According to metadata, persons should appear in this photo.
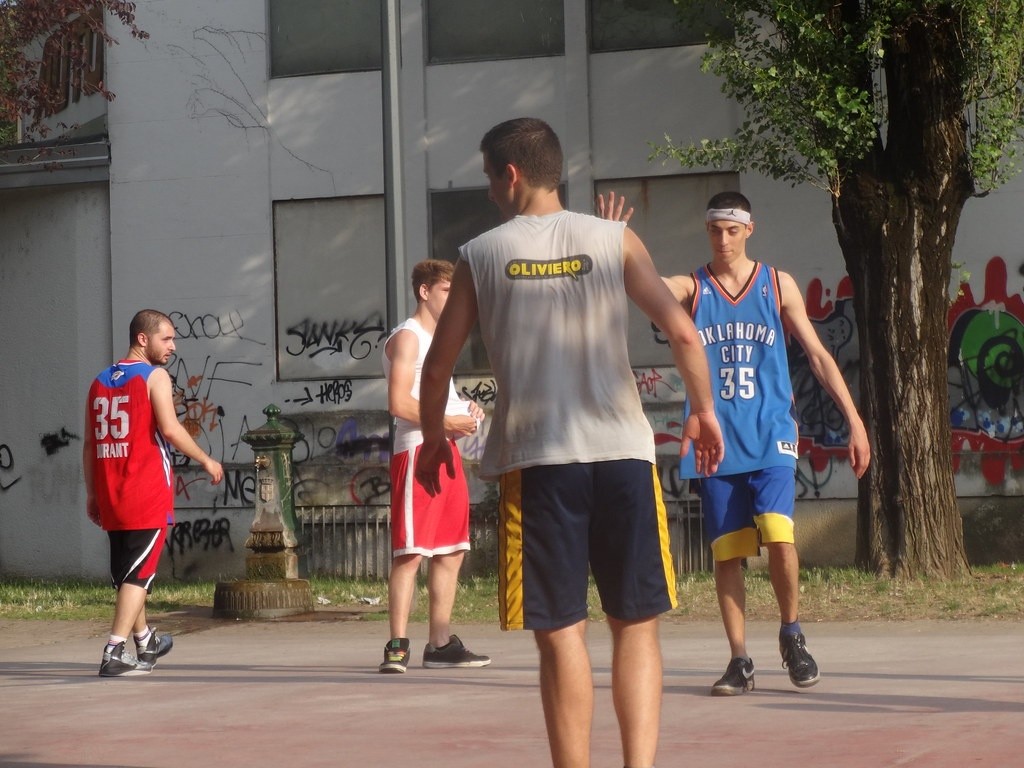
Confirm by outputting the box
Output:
[598,189,871,698]
[412,117,726,767]
[379,258,492,675]
[81,308,225,677]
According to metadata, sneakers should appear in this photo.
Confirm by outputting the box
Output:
[712,633,821,696]
[378,634,491,675]
[101,627,174,675]
